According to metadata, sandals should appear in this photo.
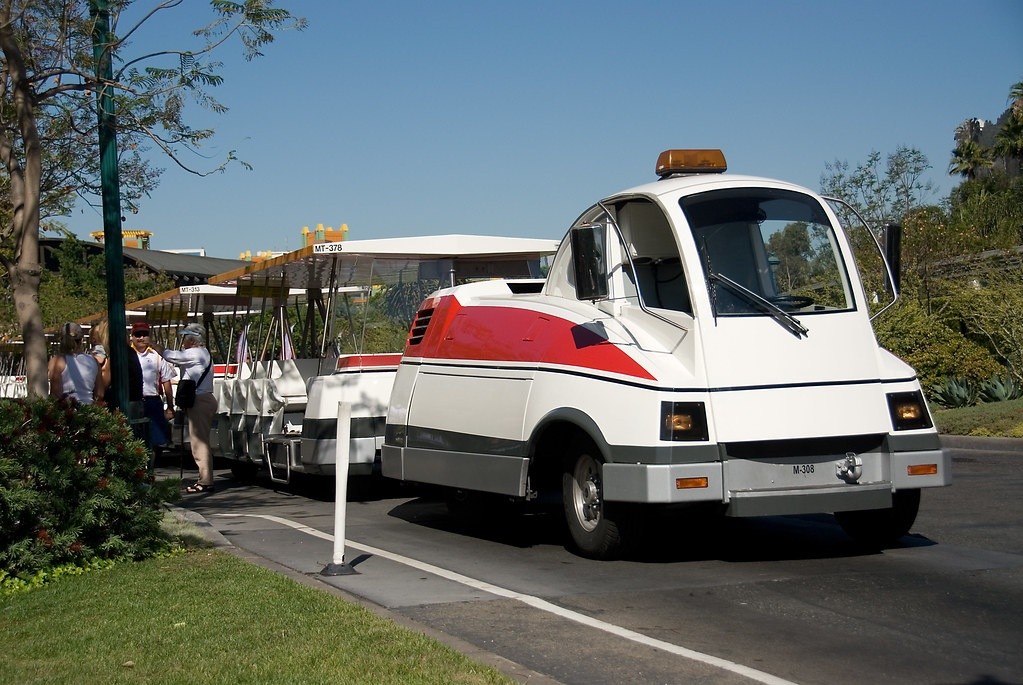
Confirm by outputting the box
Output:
[186,483,216,492]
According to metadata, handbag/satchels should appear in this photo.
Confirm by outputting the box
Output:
[175,380,196,408]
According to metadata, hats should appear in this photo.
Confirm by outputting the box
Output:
[177,322,206,337]
[63,322,83,340]
[131,322,150,337]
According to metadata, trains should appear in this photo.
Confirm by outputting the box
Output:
[0,141,954,561]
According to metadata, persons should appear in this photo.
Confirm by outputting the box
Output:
[49,321,99,408]
[132,322,177,468]
[143,322,219,493]
[88,320,143,441]
